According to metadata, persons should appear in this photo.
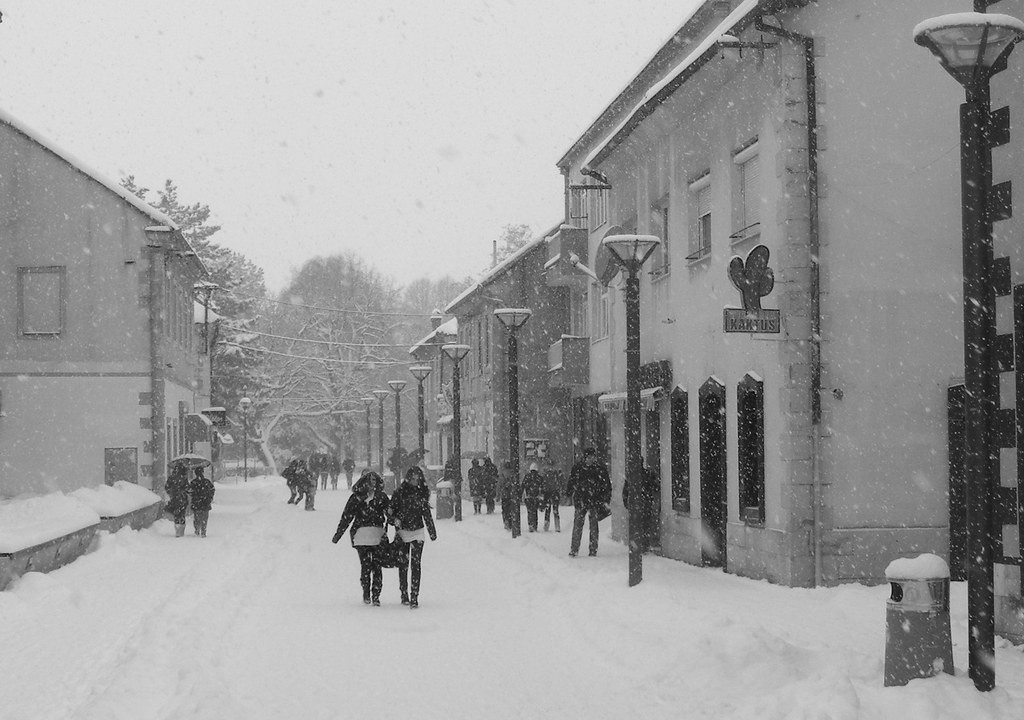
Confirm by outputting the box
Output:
[342,453,355,491]
[482,457,499,514]
[388,465,436,608]
[332,466,401,606]
[540,459,566,532]
[295,457,340,511]
[468,459,482,514]
[496,460,513,531]
[565,447,612,557]
[623,455,661,554]
[188,466,215,537]
[520,463,544,531]
[164,463,190,537]
[281,460,297,504]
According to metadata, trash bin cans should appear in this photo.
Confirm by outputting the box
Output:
[885,576,954,687]
[436,478,454,520]
[382,472,395,495]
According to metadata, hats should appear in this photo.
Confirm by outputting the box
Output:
[289,456,297,462]
[530,462,538,472]
[584,447,595,454]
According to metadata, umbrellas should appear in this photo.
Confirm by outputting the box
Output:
[166,453,211,471]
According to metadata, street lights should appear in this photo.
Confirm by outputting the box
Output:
[914,14,1024,687]
[602,235,662,585]
[373,391,389,474]
[494,309,534,539]
[442,345,470,520]
[410,367,432,474]
[388,381,407,488]
[240,397,250,482]
[360,398,374,468]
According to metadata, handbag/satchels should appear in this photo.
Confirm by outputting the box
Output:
[374,520,408,569]
[282,468,291,478]
[165,497,184,515]
[594,501,611,521]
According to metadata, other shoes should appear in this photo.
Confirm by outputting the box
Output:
[363,592,370,604]
[410,596,418,608]
[589,550,597,557]
[195,528,207,538]
[372,596,380,606]
[401,594,410,605]
[569,549,578,556]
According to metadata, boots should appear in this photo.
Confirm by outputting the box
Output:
[176,525,186,537]
